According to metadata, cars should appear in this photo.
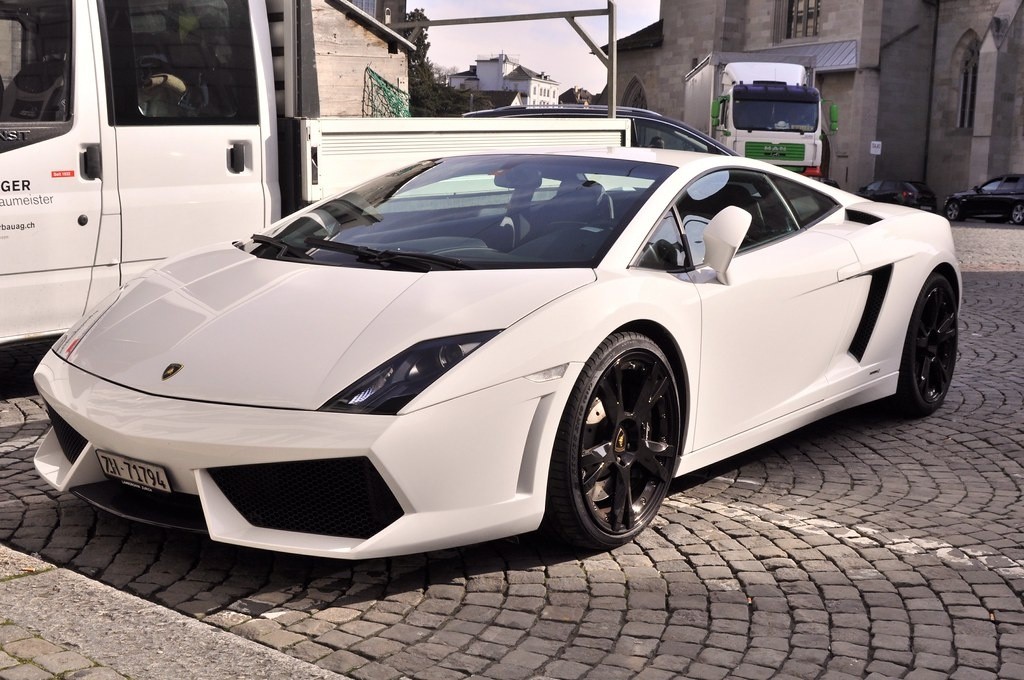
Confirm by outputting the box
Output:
[943,173,1024,224]
[853,179,938,214]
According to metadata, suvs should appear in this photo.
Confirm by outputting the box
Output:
[459,101,745,190]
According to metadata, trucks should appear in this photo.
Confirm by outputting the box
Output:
[682,51,841,190]
[0,0,633,352]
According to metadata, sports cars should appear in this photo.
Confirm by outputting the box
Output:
[31,147,963,564]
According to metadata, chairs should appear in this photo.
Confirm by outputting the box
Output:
[676,182,765,240]
[0,63,65,122]
[535,181,614,233]
